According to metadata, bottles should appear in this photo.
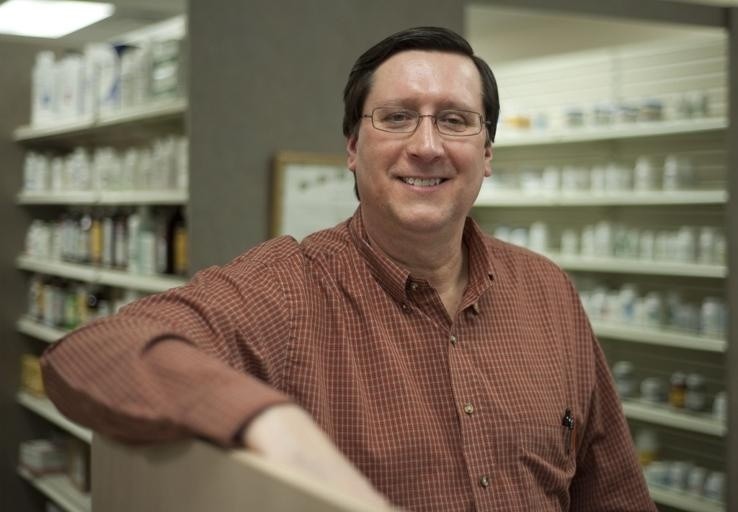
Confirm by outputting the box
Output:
[478,152,732,501]
[15,38,187,330]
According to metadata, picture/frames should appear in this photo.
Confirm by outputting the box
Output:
[269,150,358,251]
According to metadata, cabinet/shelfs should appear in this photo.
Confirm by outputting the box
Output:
[12,38,207,512]
[481,112,737,512]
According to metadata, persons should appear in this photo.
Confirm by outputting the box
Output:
[41,26,658,512]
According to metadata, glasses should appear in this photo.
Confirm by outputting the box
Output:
[359,106,492,137]
